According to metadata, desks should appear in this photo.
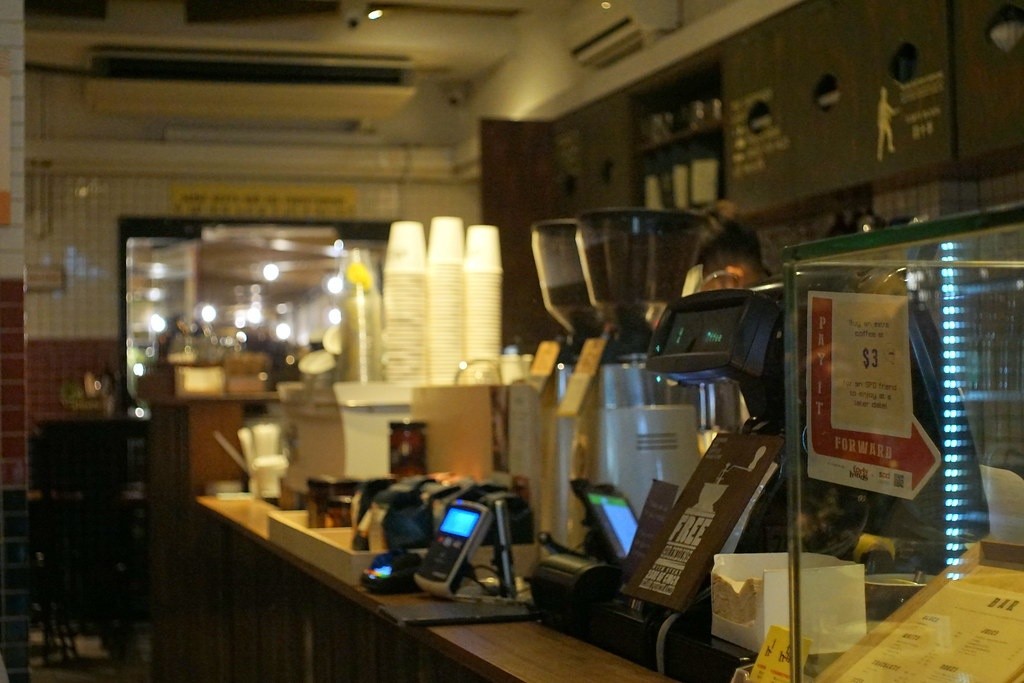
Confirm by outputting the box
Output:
[28,480,145,503]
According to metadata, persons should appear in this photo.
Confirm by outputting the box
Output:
[698,224,766,293]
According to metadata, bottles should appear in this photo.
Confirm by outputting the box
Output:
[386,422,427,478]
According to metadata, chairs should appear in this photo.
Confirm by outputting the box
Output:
[35,458,128,664]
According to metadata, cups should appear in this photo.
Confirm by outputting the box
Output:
[383,217,503,383]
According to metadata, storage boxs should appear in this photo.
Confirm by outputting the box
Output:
[782,205,1024,682]
